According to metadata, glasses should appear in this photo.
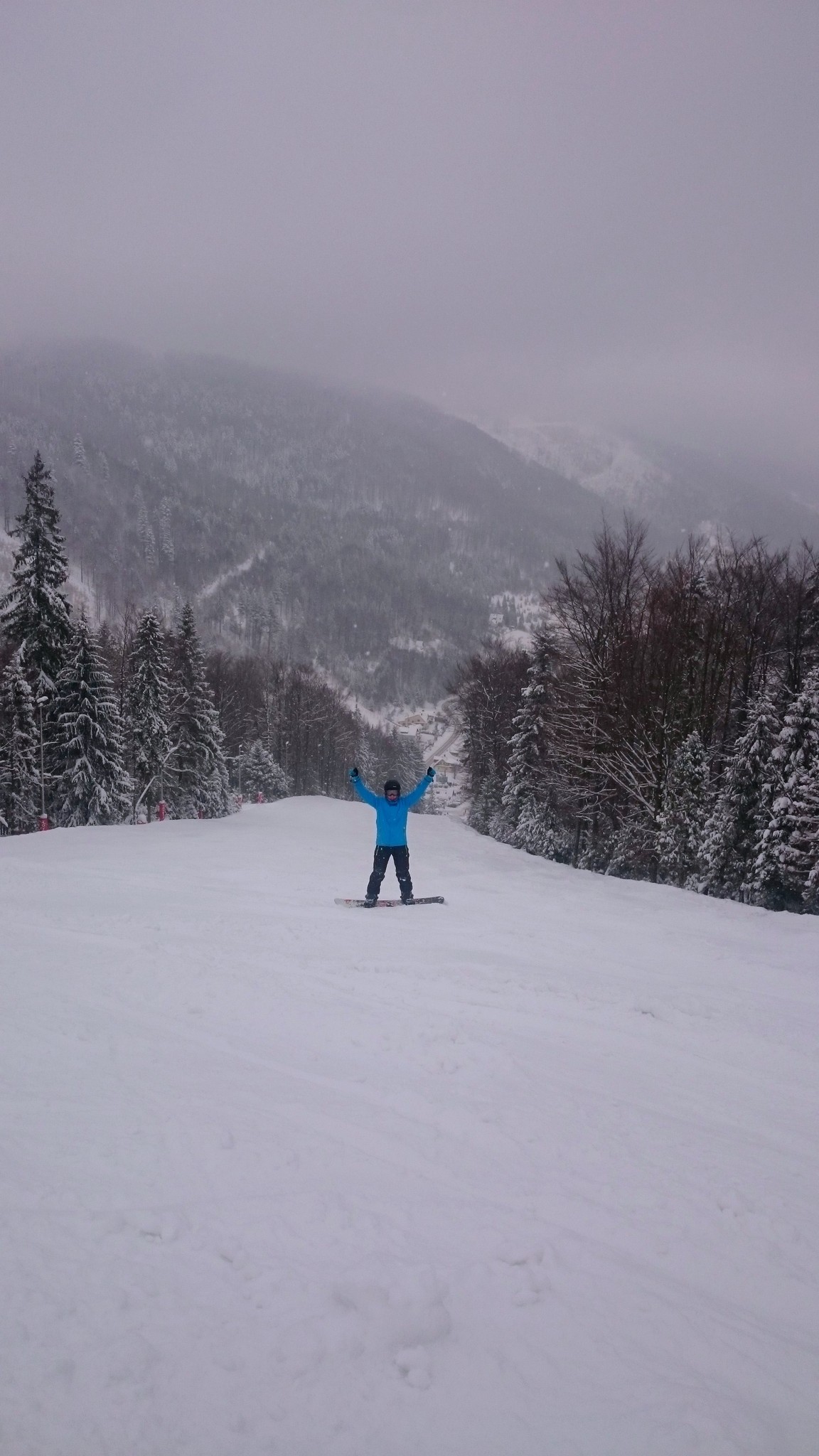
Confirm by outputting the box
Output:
[386,790,399,796]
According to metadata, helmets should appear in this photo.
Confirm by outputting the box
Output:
[384,780,400,790]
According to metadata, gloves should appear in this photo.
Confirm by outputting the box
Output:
[350,768,358,777]
[427,766,436,778]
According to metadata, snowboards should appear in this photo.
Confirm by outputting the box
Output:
[334,896,444,909]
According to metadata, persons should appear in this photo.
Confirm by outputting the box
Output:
[348,766,436,908]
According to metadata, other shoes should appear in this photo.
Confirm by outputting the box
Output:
[364,892,378,908]
[401,893,415,904]
